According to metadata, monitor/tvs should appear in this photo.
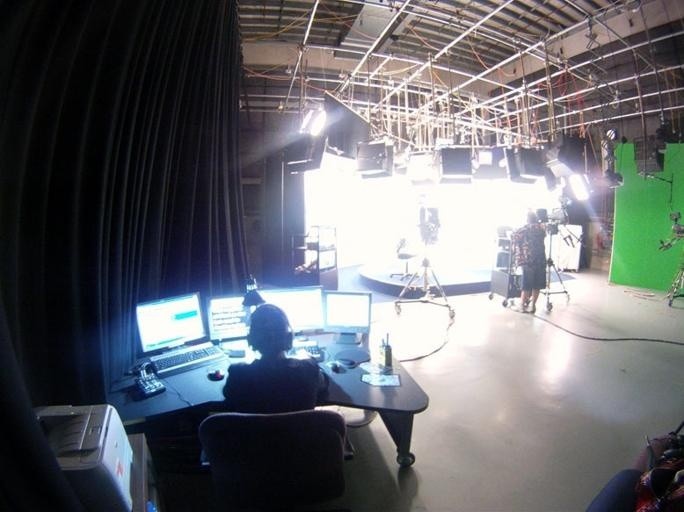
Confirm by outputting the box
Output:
[132,289,208,356]
[250,284,325,341]
[207,292,255,341]
[324,291,372,345]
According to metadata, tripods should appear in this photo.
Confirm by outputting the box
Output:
[539,231,571,312]
[392,245,457,318]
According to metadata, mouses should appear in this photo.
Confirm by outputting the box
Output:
[327,360,341,373]
[209,366,225,381]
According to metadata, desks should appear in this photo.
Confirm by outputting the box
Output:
[126,431,162,512]
[106,333,428,466]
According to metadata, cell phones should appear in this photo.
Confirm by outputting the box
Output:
[228,347,246,359]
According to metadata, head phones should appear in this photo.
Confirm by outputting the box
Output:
[245,303,293,353]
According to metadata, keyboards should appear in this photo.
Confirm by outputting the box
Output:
[148,340,224,376]
[289,343,324,363]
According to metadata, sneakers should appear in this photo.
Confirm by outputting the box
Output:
[510,305,524,312]
[524,307,534,313]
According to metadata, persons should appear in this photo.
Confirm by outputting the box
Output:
[220,303,331,413]
[509,210,549,315]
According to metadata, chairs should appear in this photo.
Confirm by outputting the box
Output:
[196,405,355,511]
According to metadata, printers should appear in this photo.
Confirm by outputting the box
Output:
[35,403,137,511]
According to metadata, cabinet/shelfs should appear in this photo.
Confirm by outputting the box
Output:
[289,224,338,292]
